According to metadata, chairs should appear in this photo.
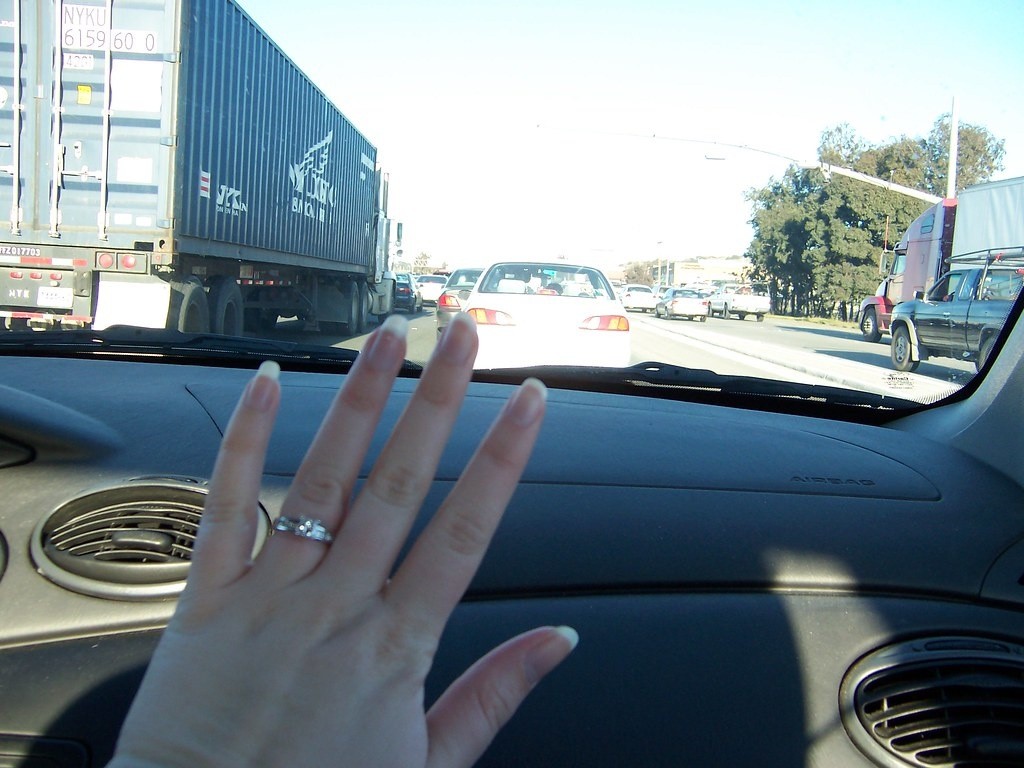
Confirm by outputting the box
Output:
[497,279,527,294]
[564,285,595,296]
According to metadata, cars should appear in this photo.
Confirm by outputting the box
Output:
[558,281,592,297]
[395,272,423,313]
[461,260,631,369]
[416,270,452,304]
[619,285,657,313]
[656,286,670,299]
[655,288,708,322]
[436,268,484,340]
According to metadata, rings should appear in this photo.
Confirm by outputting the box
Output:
[273,515,334,543]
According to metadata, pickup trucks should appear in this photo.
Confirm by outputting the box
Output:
[707,285,770,322]
[889,246,1024,373]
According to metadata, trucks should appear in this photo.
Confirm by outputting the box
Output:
[858,176,1024,343]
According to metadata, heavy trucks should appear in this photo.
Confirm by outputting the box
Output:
[0,0,402,336]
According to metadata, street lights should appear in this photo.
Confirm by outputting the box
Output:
[658,241,662,284]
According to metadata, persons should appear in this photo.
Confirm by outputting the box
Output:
[107,313,579,768]
[943,295,953,301]
[547,282,563,295]
[525,273,536,293]
